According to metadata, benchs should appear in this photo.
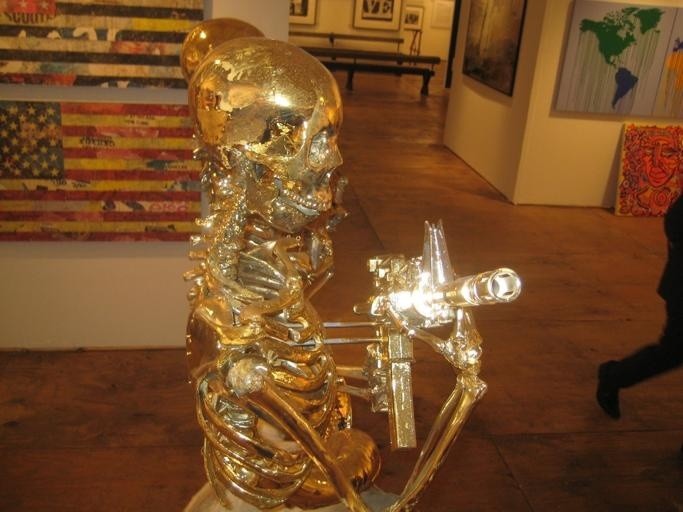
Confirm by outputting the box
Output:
[290,30,441,96]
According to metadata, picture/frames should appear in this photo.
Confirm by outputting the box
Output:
[461,0,528,97]
[289,0,318,25]
[353,0,402,31]
[404,6,424,30]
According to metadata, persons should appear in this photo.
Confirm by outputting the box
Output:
[594,192,682,417]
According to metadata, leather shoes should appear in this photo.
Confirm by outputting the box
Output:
[596,360,621,420]
